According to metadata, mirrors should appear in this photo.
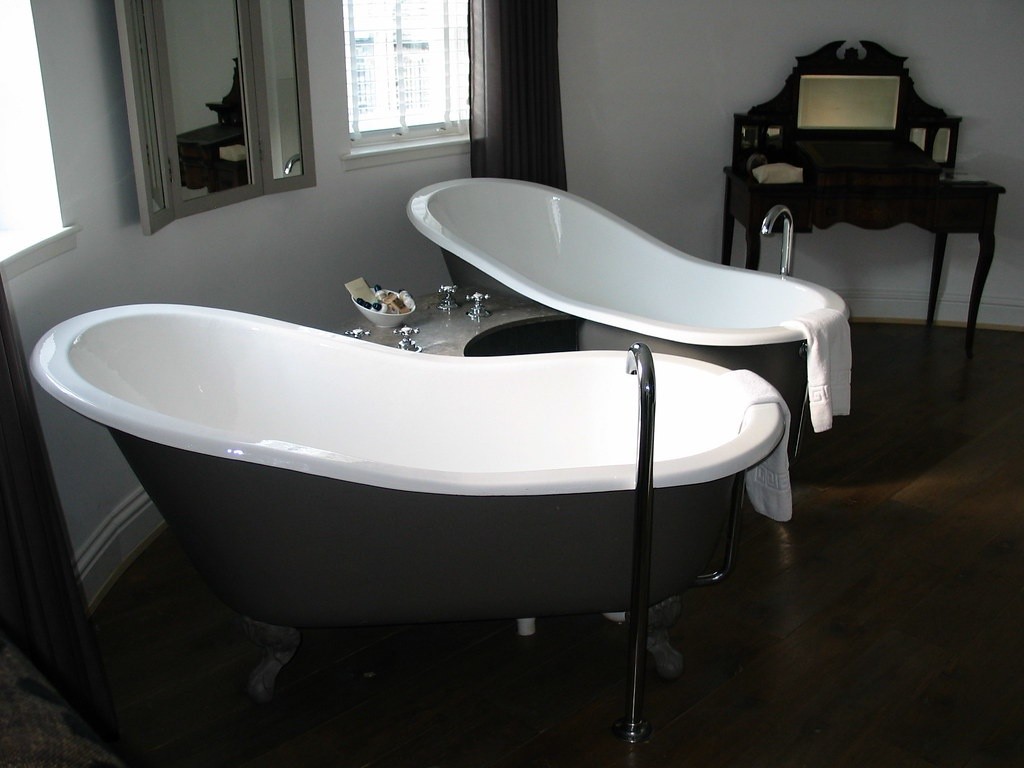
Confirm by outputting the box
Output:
[114,0,318,234]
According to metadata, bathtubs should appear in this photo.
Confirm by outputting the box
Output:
[30,305,785,707]
[406,178,852,469]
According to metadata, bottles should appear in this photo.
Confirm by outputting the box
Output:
[357,298,388,313]
[374,285,386,302]
[398,289,414,309]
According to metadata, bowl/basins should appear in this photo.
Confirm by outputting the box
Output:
[351,288,416,328]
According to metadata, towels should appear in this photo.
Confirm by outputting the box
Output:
[781,306,853,432]
[685,369,794,521]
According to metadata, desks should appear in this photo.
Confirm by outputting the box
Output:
[721,39,1007,361]
[171,54,251,196]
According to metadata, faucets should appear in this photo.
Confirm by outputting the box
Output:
[613,341,651,742]
[762,206,794,279]
